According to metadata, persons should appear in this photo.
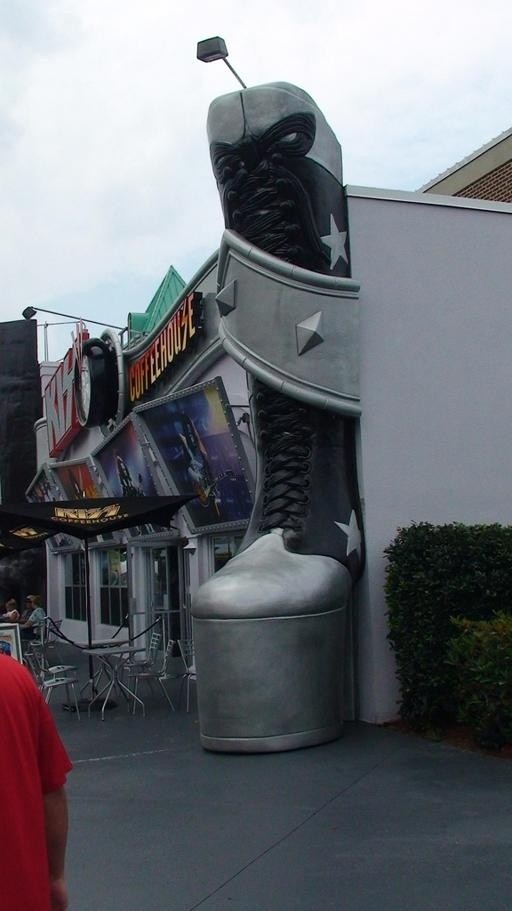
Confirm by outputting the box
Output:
[1,593,47,651]
[0,648,72,908]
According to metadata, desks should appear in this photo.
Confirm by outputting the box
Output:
[0,617,40,630]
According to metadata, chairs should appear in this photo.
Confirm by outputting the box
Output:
[24,619,197,722]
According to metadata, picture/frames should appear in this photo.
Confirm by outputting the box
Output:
[0,623,23,665]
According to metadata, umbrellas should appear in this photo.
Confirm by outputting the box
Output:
[0,488,192,718]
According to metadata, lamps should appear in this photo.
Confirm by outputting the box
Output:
[22,305,123,331]
[197,36,245,86]
